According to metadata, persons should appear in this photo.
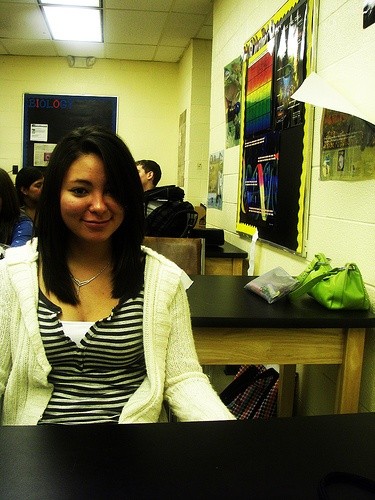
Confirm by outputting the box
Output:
[0,124,238,426]
[0,166,45,260]
[135,159,184,238]
[244,19,276,62]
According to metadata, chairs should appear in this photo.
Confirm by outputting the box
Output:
[141,237,205,275]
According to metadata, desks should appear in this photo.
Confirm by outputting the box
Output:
[197,236,248,275]
[0,413,375,500]
[186,276,375,414]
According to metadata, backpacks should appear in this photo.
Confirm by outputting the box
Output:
[142,201,199,238]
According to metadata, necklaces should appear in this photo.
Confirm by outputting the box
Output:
[68,258,111,295]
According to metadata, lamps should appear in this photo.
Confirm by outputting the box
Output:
[66,55,96,66]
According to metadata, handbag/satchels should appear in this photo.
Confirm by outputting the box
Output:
[219,363,301,420]
[288,252,371,310]
